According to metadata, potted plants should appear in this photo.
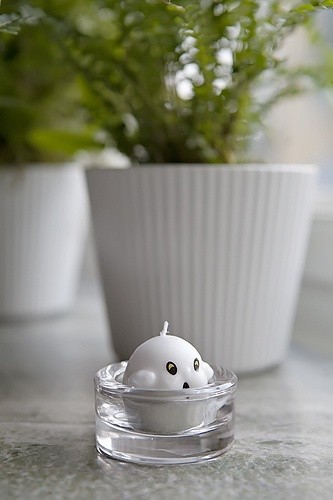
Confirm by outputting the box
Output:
[0,0,186,320]
[0,0,333,374]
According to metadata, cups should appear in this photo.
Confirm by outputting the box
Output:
[85,164,318,375]
[0,161,91,323]
